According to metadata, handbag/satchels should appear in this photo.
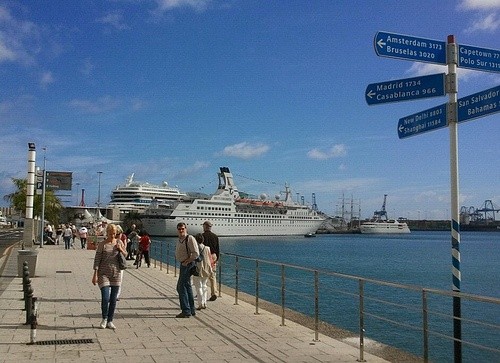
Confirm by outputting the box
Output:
[190,266,199,276]
[118,250,127,270]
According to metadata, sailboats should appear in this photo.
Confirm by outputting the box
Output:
[315,193,365,234]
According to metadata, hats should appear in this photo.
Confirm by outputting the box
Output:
[202,221,212,226]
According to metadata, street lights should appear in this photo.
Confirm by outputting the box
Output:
[96,171,103,207]
[40,146,47,249]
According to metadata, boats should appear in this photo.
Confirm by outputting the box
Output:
[251,198,264,206]
[264,200,276,207]
[359,219,411,234]
[234,199,251,205]
[304,232,316,237]
[276,201,285,208]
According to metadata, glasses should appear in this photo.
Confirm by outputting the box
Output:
[178,228,185,230]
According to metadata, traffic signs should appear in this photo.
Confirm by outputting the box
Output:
[397,101,449,140]
[365,71,447,106]
[373,30,448,66]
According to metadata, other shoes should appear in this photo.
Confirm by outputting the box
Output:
[176,313,189,317]
[107,322,116,329]
[116,298,119,301]
[197,305,206,310]
[100,319,107,328]
[210,296,216,301]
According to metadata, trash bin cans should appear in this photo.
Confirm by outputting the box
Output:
[16,250,39,278]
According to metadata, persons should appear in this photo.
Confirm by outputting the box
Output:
[175,223,199,318]
[121,224,150,269]
[99,222,107,227]
[45,222,77,250]
[92,224,127,329]
[109,225,123,300]
[203,221,220,301]
[194,234,214,310]
[79,224,88,248]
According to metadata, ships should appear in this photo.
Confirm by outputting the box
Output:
[106,173,186,214]
[126,167,326,236]
